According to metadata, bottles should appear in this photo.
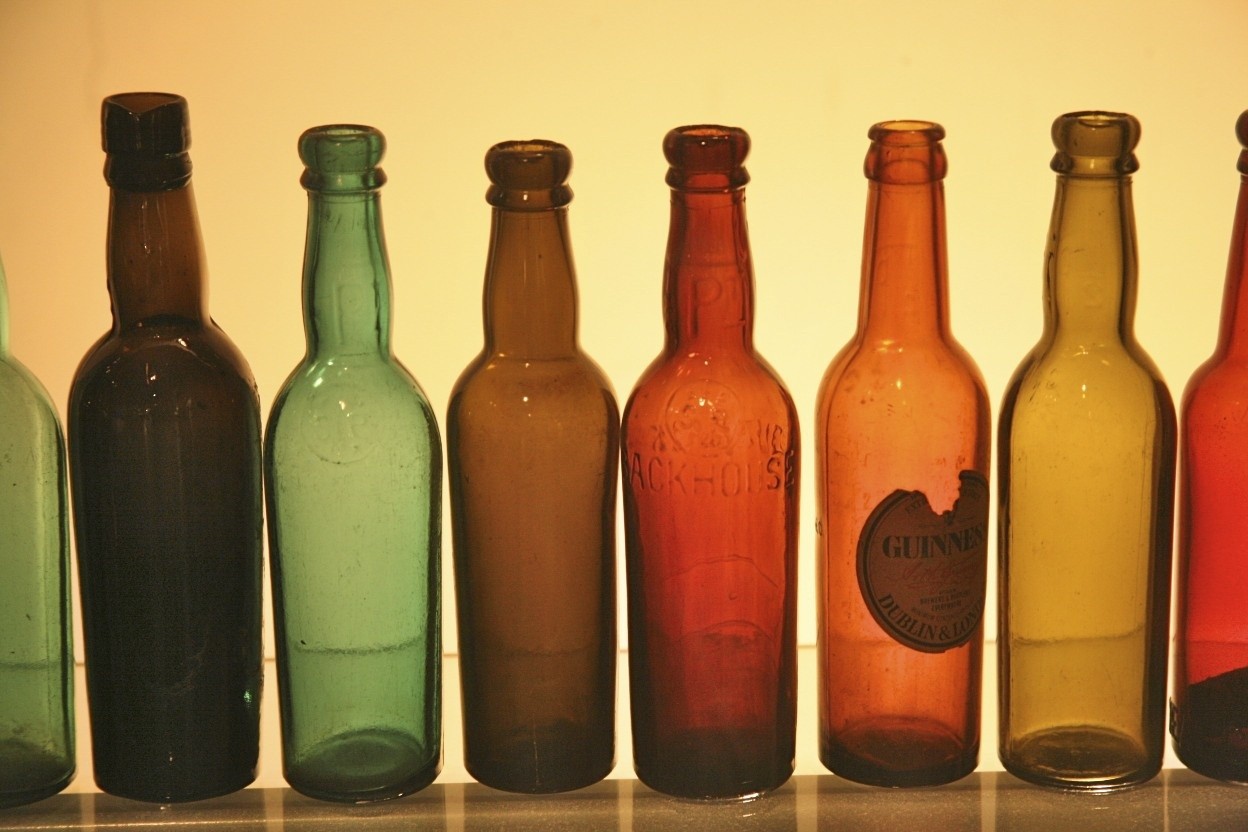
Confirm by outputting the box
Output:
[1169,109,1247,790]
[812,117,991,792]
[0,256,74,809]
[446,139,622,794]
[66,95,264,806]
[622,123,803,803]
[996,109,1178,798]
[264,122,444,809]
